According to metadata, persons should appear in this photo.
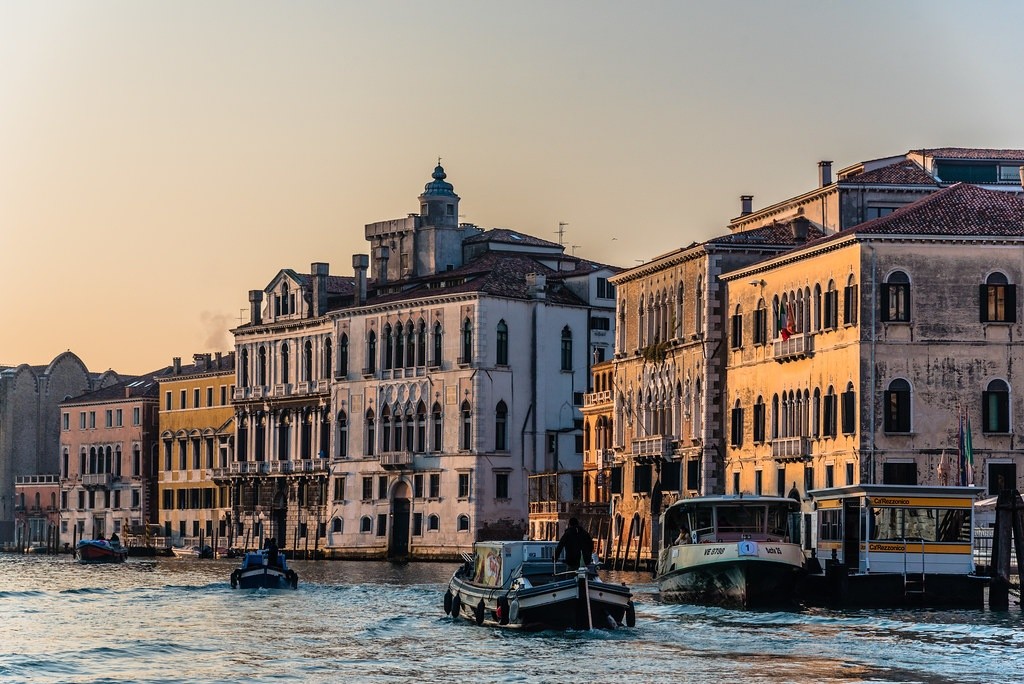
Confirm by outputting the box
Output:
[95,532,105,540]
[108,532,119,541]
[673,525,692,546]
[261,538,279,565]
[552,517,594,580]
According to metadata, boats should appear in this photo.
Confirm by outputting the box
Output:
[231,548,298,590]
[77,540,128,564]
[24,544,47,555]
[443,540,635,636]
[649,492,803,613]
[171,546,237,560]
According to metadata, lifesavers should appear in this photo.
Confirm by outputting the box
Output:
[496,595,509,625]
[230,569,241,587]
[286,569,298,589]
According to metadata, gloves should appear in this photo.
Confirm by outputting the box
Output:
[551,556,558,563]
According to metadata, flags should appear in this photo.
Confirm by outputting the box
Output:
[780,303,796,342]
[958,410,975,486]
